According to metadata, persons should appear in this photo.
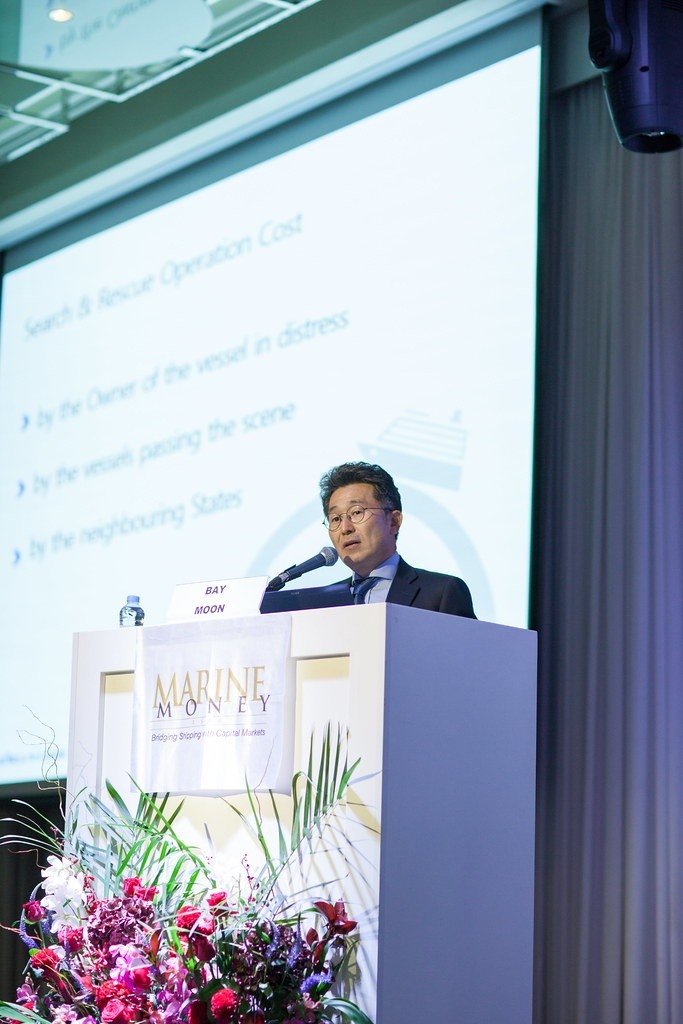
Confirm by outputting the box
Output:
[308,464,474,617]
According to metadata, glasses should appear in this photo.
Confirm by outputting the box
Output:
[322,505,394,531]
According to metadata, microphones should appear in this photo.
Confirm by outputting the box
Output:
[270,547,338,587]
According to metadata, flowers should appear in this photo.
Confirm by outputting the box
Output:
[0,701,378,1024]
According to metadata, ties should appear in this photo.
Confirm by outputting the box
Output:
[352,576,379,604]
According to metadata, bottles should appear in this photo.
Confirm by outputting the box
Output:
[119,595,145,628]
